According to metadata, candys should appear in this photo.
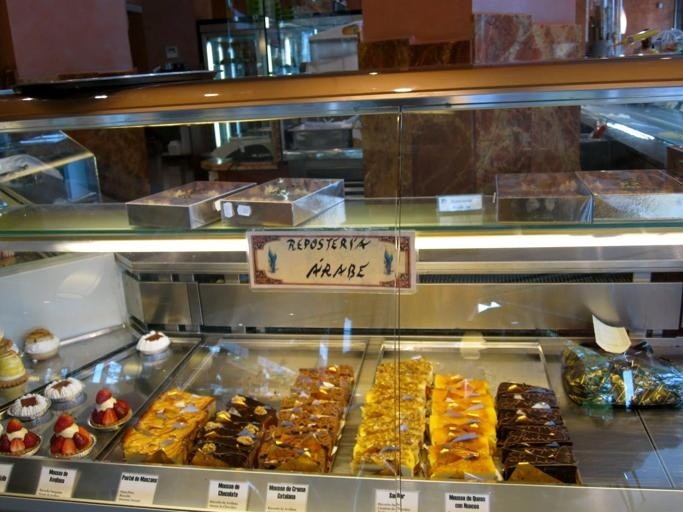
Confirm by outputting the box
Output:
[564,341,683,411]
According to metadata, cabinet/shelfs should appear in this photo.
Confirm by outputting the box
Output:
[0,54,681,512]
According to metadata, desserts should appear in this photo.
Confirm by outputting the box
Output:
[1,329,577,488]
